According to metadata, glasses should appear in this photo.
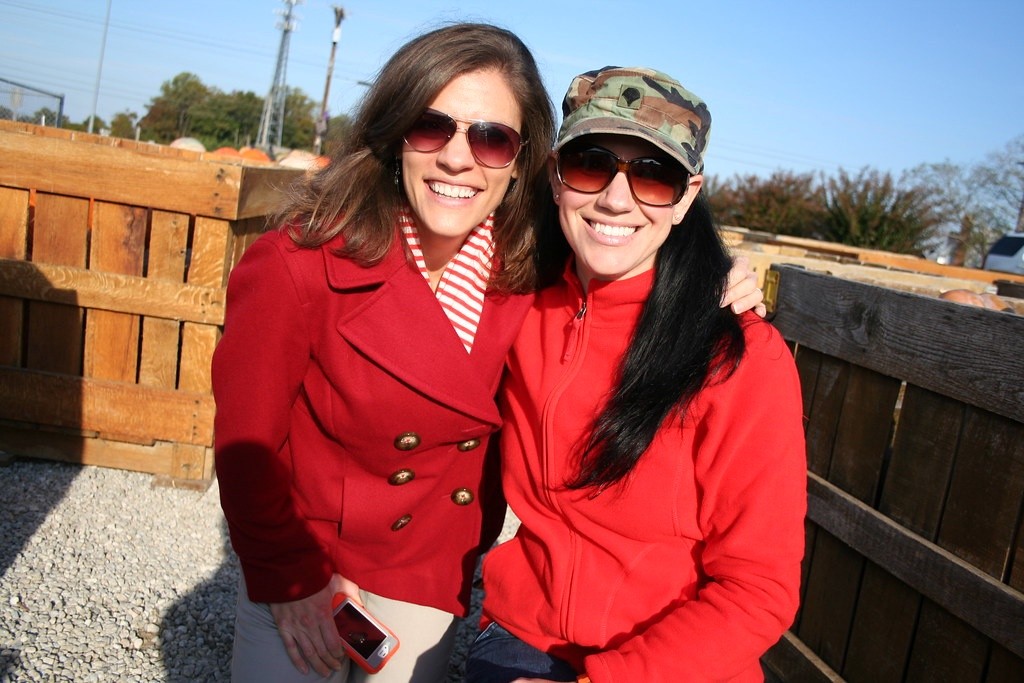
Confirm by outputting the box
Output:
[402,108,528,167]
[556,143,689,207]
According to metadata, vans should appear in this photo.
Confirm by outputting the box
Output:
[981,233,1024,275]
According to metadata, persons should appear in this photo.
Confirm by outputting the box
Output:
[445,67,807,683]
[210,26,766,683]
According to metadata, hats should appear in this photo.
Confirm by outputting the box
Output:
[552,66,711,175]
